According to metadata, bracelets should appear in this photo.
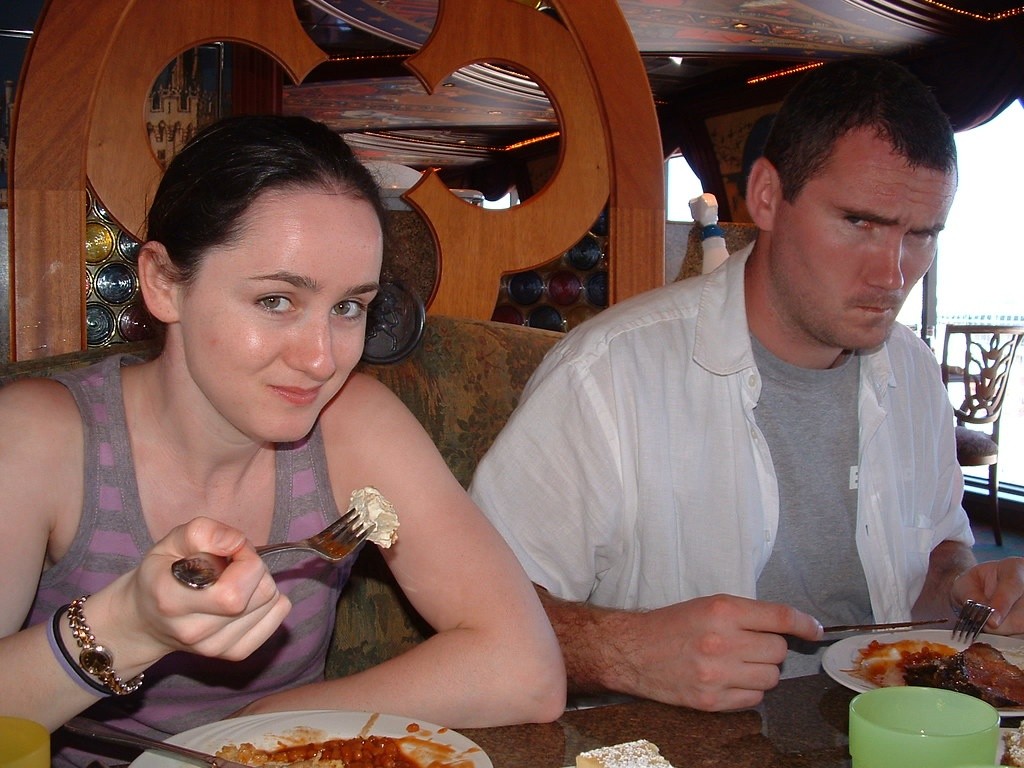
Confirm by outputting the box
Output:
[45,611,101,695]
[53,603,114,695]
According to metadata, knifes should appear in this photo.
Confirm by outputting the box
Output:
[823,618,949,633]
[66,715,251,768]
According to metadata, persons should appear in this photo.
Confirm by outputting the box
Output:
[0,110,566,768]
[464,57,1024,714]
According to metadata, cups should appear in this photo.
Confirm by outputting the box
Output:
[0,715,52,768]
[848,686,1001,768]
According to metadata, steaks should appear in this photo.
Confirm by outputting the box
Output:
[933,642,1024,709]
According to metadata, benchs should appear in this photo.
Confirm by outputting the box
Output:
[0,279,569,703]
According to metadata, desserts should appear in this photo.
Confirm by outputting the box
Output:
[575,738,674,768]
[345,484,400,550]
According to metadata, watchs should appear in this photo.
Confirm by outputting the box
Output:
[68,594,144,696]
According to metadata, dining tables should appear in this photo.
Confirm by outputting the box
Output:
[444,632,1024,768]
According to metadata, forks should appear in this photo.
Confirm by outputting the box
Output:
[171,508,376,591]
[951,598,996,641]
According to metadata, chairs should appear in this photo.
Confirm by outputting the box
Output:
[941,324,1024,547]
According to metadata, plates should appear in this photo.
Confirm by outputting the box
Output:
[821,629,1024,717]
[126,709,494,768]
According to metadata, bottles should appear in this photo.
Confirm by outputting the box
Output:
[699,225,730,275]
